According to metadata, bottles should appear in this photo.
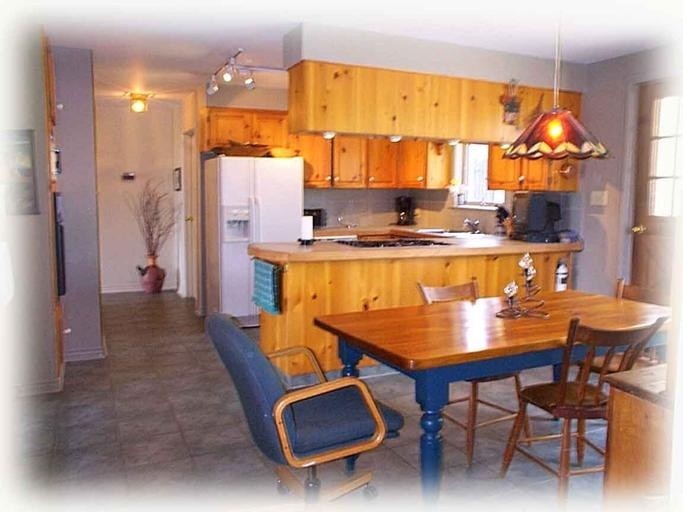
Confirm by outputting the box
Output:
[555,261,568,291]
[458,191,465,205]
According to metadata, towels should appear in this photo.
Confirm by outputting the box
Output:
[251,258,281,315]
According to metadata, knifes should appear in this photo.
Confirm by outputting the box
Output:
[495,206,508,224]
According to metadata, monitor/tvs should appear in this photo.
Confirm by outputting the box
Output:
[509,192,563,233]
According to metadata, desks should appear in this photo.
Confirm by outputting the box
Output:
[312,289,671,512]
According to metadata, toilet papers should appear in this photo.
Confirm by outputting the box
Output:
[299,215,314,241]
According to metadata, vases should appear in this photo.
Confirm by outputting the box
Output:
[137,255,165,292]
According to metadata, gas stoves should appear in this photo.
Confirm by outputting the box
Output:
[335,240,453,250]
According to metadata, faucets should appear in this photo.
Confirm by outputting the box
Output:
[464,219,480,231]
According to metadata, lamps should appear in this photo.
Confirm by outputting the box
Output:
[502,23,608,161]
[206,42,286,93]
[124,91,153,113]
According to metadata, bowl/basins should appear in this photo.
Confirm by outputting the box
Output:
[201,139,299,159]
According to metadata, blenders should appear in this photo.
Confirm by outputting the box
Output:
[394,194,414,226]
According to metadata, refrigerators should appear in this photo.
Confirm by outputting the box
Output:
[203,157,304,329]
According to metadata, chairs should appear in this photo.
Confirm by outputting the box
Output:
[501,316,670,501]
[577,277,668,381]
[207,311,404,502]
[415,276,532,468]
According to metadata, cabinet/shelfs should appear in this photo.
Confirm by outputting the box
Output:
[288,62,583,196]
[200,104,287,156]
